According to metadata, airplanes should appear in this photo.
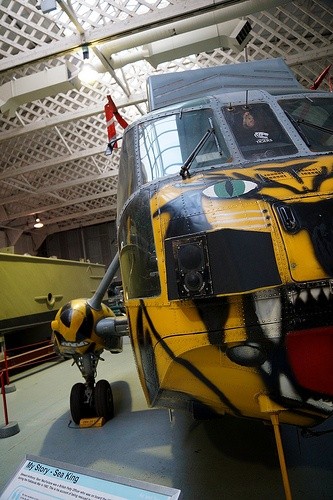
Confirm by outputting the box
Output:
[48,52,332,444]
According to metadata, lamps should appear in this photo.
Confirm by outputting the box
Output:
[33,214,44,229]
